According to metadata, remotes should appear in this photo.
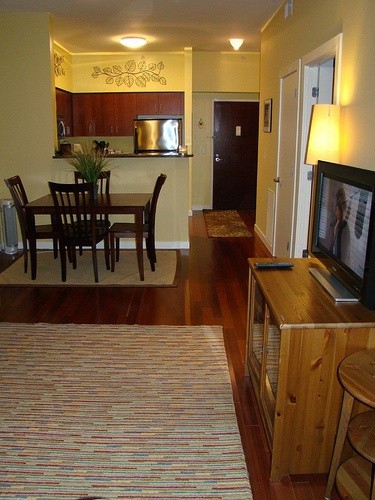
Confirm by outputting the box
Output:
[254,261,293,268]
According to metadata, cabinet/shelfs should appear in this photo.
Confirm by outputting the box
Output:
[71,94,95,137]
[95,93,136,136]
[245,256,375,483]
[138,92,184,136]
[324,350,374,500]
[56,90,71,137]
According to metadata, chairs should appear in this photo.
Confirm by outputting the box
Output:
[45,180,110,284]
[4,175,63,273]
[110,173,168,280]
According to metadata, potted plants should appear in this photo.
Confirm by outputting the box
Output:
[64,145,113,199]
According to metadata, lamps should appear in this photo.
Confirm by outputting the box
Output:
[230,39,244,52]
[305,103,340,166]
[118,37,147,50]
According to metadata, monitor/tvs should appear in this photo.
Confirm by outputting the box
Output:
[307,160,375,312]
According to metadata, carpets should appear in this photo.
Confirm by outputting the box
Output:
[0,251,182,290]
[0,322,256,499]
[203,208,254,238]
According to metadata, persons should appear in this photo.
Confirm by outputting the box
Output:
[327,190,351,264]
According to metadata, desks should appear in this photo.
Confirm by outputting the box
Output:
[22,193,154,282]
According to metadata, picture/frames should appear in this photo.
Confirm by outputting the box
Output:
[262,98,272,133]
